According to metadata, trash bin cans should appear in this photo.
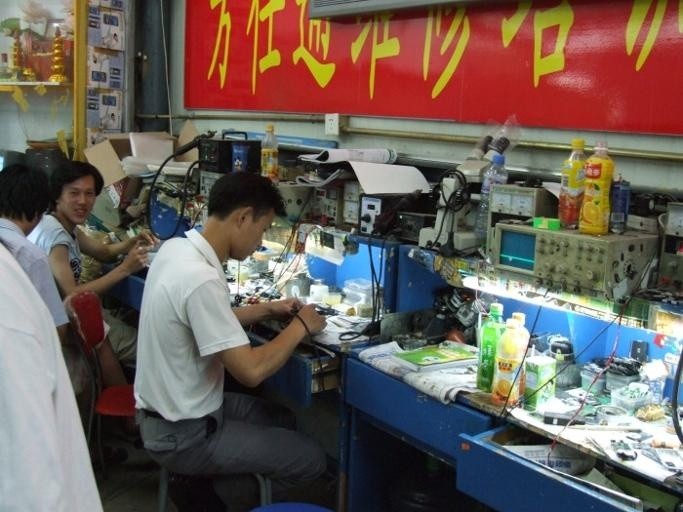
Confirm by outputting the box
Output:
[626,432,641,439]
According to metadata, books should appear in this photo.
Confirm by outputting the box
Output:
[391,342,478,372]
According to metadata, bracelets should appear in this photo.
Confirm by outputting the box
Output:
[294,313,312,337]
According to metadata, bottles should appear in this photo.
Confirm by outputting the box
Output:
[260,125,279,185]
[476,302,531,409]
[557,138,614,235]
[474,155,509,244]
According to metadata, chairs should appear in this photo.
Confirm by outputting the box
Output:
[66,288,137,449]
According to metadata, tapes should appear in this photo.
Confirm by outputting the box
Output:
[532,217,562,231]
[596,404,629,423]
[325,294,341,306]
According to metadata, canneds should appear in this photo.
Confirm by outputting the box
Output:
[610,181,631,232]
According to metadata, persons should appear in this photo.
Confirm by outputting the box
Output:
[133,171,328,512]
[0,163,70,341]
[24,162,157,464]
[0,242,104,512]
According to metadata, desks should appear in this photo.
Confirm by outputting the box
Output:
[348,245,681,508]
[98,198,442,509]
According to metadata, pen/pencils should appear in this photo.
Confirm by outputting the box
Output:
[571,426,642,430]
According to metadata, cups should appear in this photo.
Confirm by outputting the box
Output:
[549,353,576,386]
